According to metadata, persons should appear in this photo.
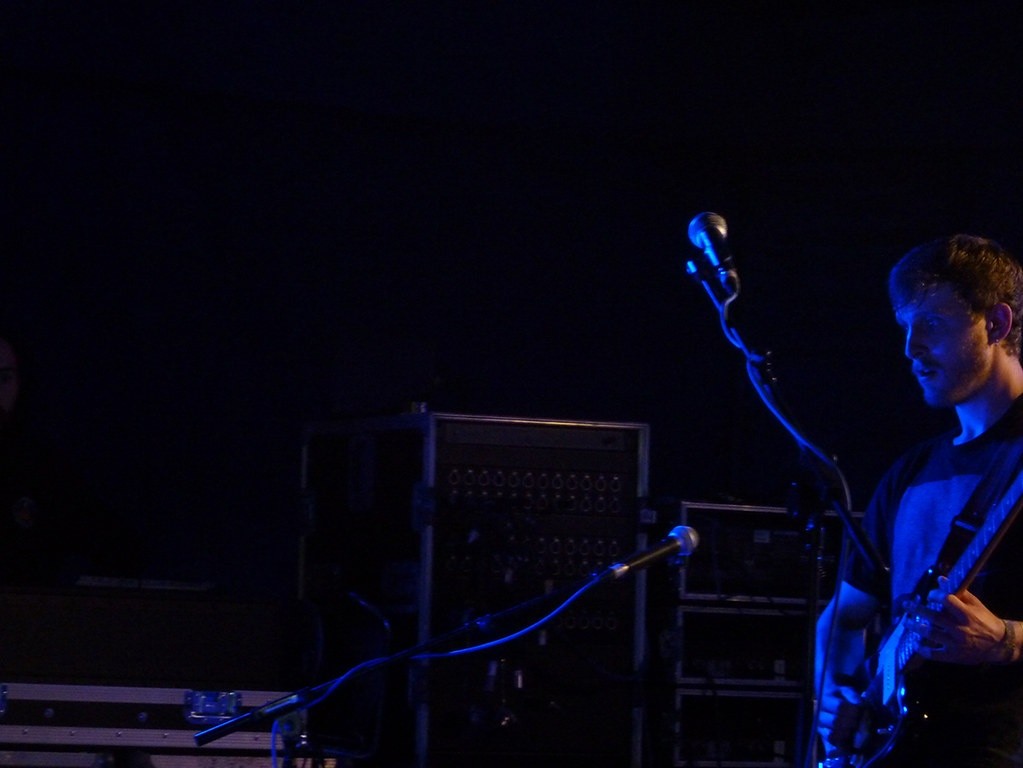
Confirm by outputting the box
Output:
[813,234,1023,767]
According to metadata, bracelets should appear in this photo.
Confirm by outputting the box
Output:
[999,617,1016,663]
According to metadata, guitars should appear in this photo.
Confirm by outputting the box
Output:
[819,470,1023,768]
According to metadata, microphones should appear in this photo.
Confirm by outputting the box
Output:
[688,212,739,296]
[601,526,699,583]
